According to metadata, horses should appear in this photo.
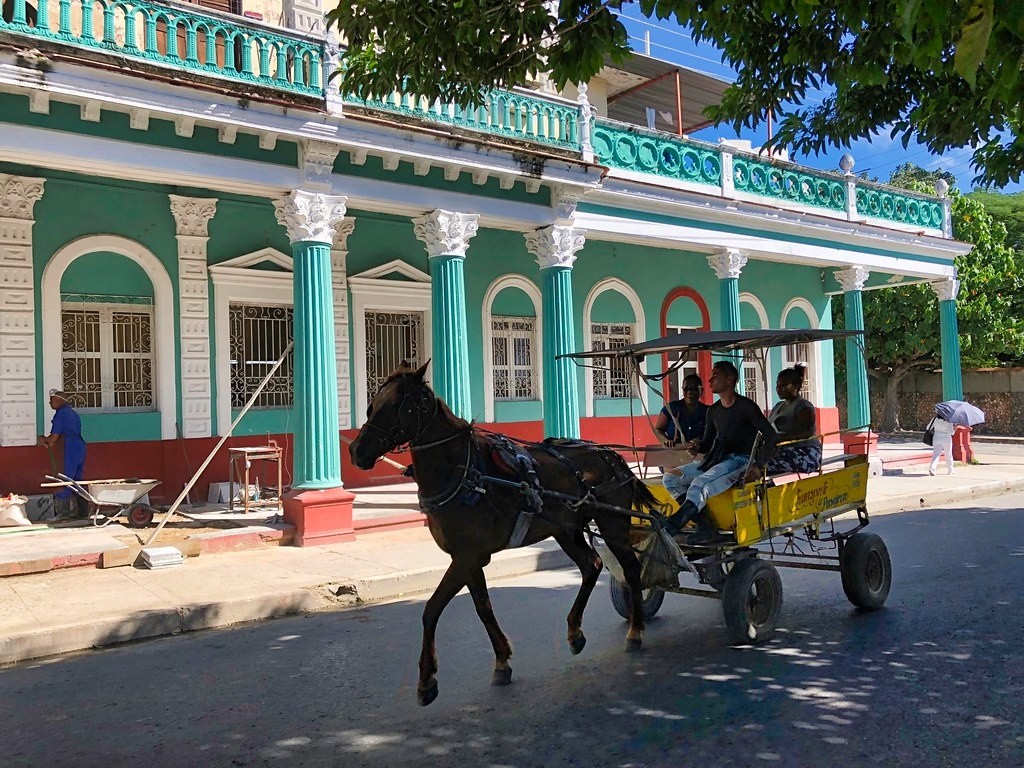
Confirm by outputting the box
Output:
[347,359,665,708]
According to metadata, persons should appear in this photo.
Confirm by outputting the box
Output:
[926,413,957,477]
[654,374,713,476]
[38,391,85,523]
[648,359,779,546]
[757,361,823,476]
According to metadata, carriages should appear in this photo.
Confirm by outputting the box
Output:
[347,327,895,708]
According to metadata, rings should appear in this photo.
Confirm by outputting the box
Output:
[666,441,668,443]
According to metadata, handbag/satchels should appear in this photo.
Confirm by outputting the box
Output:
[696,435,726,472]
[923,417,936,446]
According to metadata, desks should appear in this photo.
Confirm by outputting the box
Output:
[228,447,284,514]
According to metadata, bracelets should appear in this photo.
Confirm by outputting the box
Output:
[690,440,695,444]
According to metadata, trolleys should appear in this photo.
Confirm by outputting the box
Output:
[44,473,163,529]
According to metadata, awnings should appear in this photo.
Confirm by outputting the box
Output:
[601,46,773,159]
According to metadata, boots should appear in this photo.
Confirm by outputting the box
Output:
[46,497,70,521]
[70,494,89,518]
[675,492,721,545]
[649,499,699,537]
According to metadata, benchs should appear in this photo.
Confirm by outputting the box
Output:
[638,444,762,531]
[756,453,857,483]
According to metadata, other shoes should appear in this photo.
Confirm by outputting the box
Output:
[929,469,935,476]
[948,472,957,476]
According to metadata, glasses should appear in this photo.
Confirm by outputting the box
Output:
[682,386,703,391]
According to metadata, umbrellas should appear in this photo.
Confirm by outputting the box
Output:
[934,399,986,428]
